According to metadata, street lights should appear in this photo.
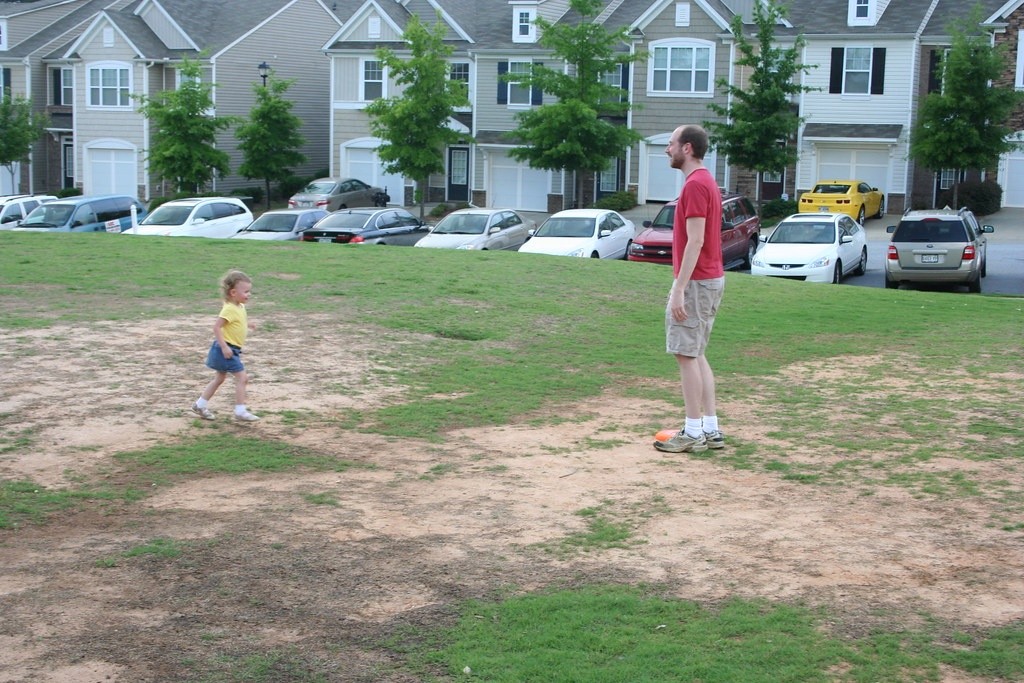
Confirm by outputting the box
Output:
[258,61,271,210]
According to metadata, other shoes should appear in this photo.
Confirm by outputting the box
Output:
[235,412,259,422]
[190,403,214,420]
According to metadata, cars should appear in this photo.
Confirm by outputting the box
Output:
[751,213,867,285]
[118,197,255,239]
[798,179,884,226]
[288,175,386,212]
[414,207,537,252]
[230,205,332,241]
[517,208,637,260]
[296,207,436,246]
[10,193,148,234]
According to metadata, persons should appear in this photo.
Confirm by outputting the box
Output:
[653,125,725,454]
[192,271,261,422]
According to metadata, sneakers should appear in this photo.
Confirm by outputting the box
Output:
[701,429,724,449]
[653,428,708,453]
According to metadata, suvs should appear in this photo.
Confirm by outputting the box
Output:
[1,195,60,229]
[884,207,995,294]
[627,187,762,272]
